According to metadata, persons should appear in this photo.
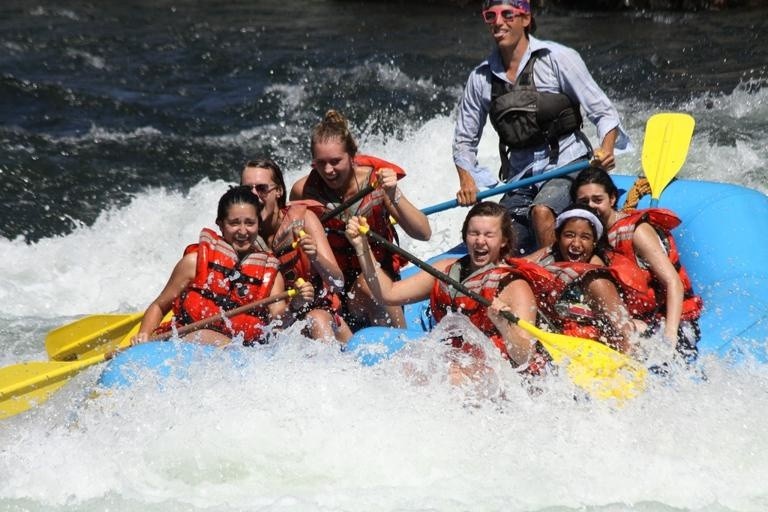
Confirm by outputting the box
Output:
[346,199,542,386]
[289,109,433,342]
[520,164,704,375]
[450,0,633,253]
[240,158,343,348]
[521,199,634,368]
[129,187,315,354]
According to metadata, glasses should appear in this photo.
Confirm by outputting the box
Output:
[242,181,283,194]
[482,8,530,24]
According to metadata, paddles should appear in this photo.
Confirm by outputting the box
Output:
[44,231,310,361]
[358,226,651,410]
[1,277,306,420]
[642,113,695,209]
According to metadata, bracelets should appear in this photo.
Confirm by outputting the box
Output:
[354,244,372,258]
[392,192,402,203]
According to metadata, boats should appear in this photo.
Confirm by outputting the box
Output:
[70,174,767,429]
